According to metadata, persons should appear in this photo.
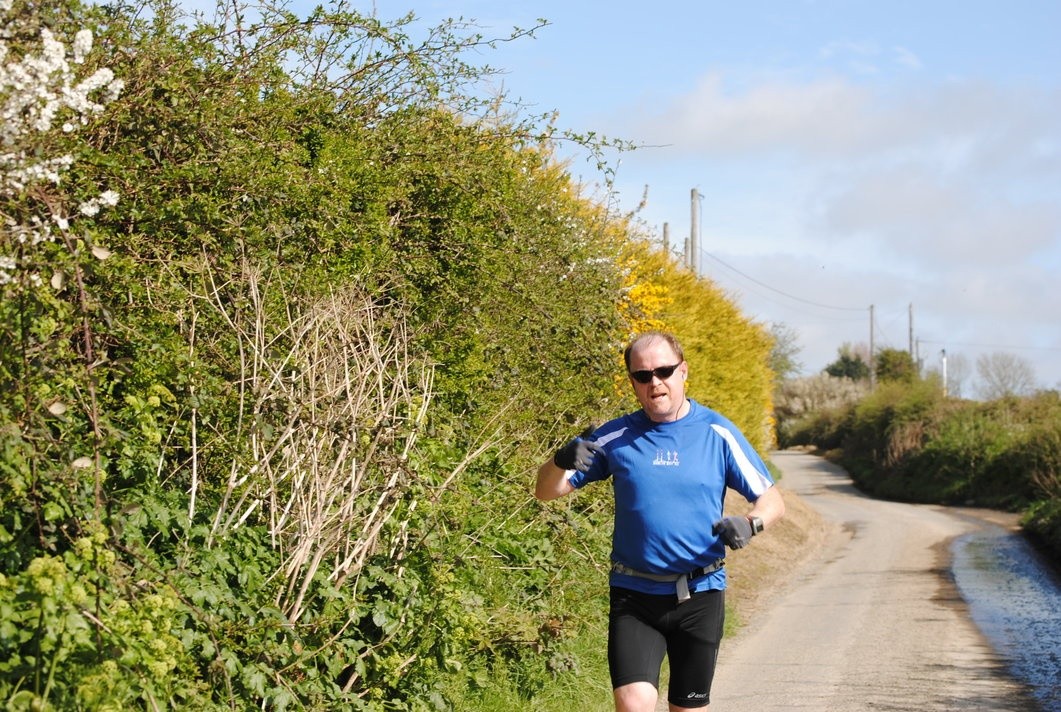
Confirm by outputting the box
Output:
[536,331,785,712]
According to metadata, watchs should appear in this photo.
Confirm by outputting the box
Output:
[746,515,765,538]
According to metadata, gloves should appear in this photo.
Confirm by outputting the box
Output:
[554,424,609,474]
[712,516,754,550]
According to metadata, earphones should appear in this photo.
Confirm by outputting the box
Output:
[681,370,686,380]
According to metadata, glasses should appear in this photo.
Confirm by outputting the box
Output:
[631,361,683,383]
